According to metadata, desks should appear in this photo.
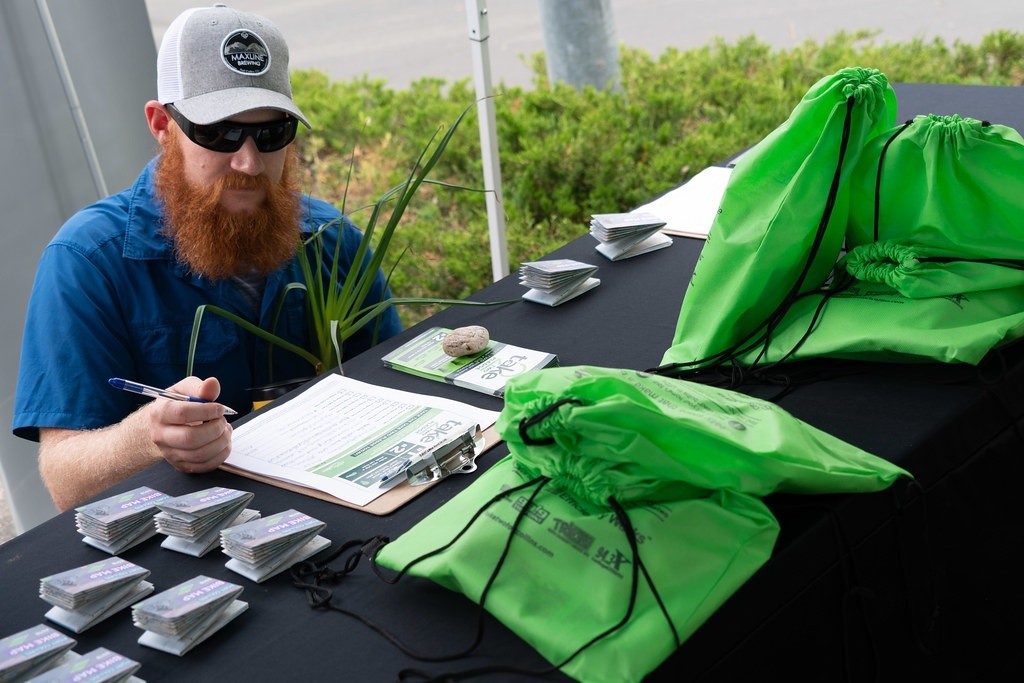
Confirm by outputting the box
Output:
[0,80,1024,681]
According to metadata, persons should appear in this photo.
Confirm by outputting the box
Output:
[13,4,403,512]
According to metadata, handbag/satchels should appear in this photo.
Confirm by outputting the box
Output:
[655,67,1024,366]
[495,364,914,508]
[368,451,779,683]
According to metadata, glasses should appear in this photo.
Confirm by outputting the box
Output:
[166,103,298,153]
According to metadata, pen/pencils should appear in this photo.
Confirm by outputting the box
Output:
[108,376,239,423]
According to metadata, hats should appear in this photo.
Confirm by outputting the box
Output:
[157,3,312,129]
